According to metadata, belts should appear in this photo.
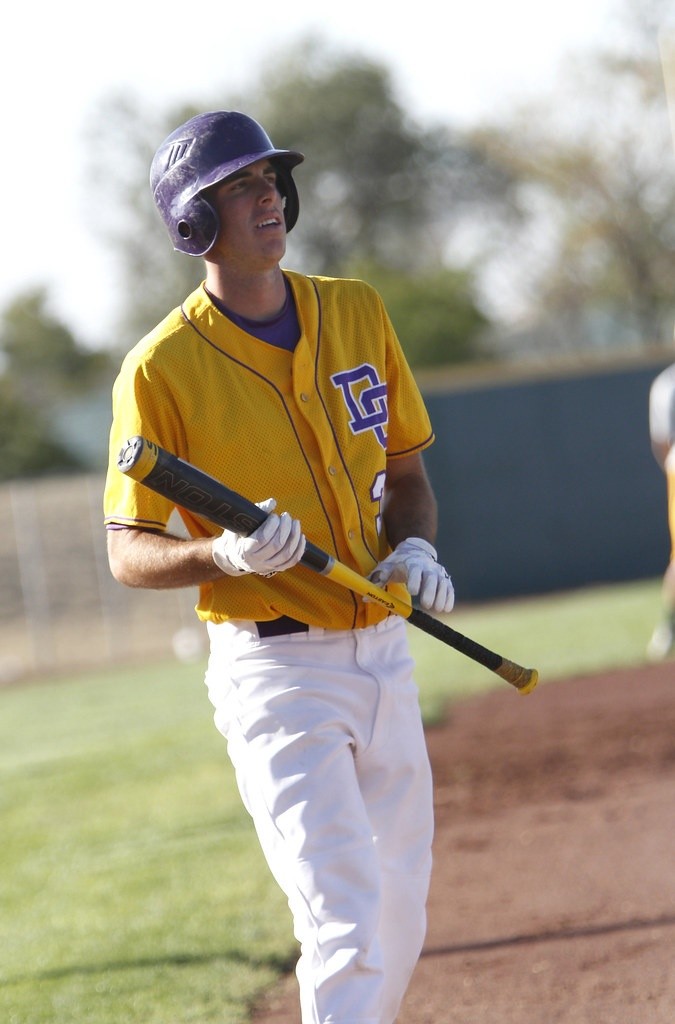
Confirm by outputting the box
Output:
[255,615,309,638]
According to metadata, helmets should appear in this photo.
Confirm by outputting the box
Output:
[150,111,304,257]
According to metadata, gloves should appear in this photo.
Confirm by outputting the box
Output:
[212,498,306,578]
[362,537,454,612]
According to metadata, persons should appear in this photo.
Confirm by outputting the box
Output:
[102,109,456,1024]
[645,366,674,654]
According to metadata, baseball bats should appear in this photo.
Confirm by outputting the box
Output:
[116,435,538,696]
